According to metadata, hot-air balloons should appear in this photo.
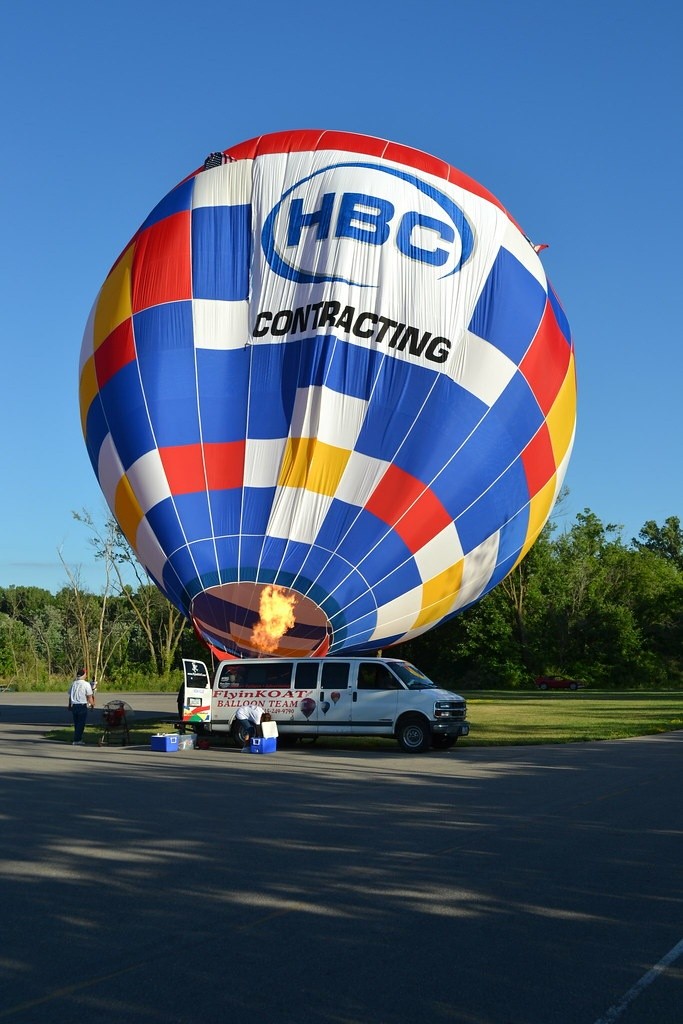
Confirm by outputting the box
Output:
[79,128,579,735]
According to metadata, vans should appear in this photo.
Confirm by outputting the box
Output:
[180,657,470,753]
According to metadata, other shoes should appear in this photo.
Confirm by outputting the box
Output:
[241,746,251,753]
[73,741,85,745]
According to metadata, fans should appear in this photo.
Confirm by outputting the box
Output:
[99,700,136,747]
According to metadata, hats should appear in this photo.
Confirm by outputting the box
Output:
[77,670,85,677]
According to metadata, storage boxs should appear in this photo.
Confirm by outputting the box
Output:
[179,734,198,750]
[251,721,279,754]
[149,733,179,752]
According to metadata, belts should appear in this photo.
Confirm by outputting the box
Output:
[73,704,86,706]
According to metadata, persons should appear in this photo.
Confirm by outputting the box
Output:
[235,705,272,753]
[177,682,187,735]
[67,669,95,745]
[90,676,97,697]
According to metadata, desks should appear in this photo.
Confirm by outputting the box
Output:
[161,720,209,733]
